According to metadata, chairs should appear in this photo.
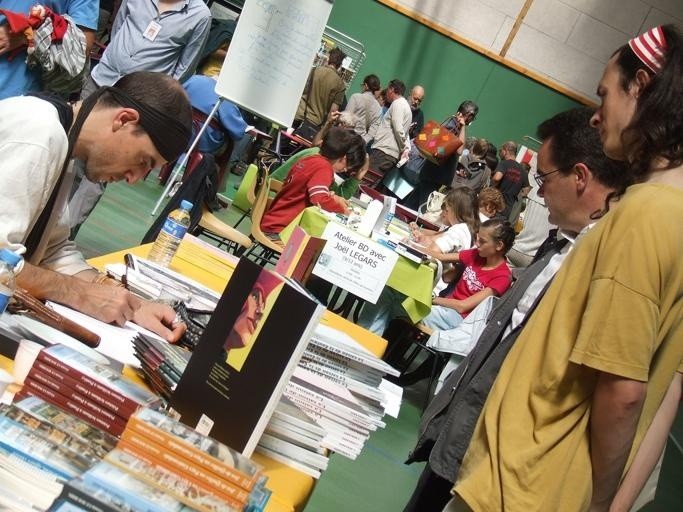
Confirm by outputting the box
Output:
[188,151,251,258]
[388,263,516,414]
[238,168,285,267]
[218,181,283,255]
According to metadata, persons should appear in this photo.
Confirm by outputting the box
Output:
[444,23,683,511]
[449,137,491,194]
[345,74,382,145]
[507,161,531,226]
[179,70,257,214]
[0,0,99,101]
[477,187,504,223]
[406,85,425,139]
[66,1,213,246]
[366,89,390,155]
[491,141,530,219]
[257,129,370,200]
[220,283,268,361]
[361,79,412,189]
[312,109,366,146]
[486,143,498,170]
[403,99,479,214]
[0,70,193,343]
[411,186,479,296]
[505,184,557,272]
[408,201,452,236]
[405,105,636,511]
[356,217,513,337]
[458,136,476,163]
[197,13,240,77]
[260,128,356,251]
[273,46,345,152]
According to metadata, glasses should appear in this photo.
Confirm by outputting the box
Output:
[533,163,573,187]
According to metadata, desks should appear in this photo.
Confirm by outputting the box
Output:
[306,198,432,332]
[0,235,389,511]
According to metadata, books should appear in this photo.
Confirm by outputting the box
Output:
[371,231,431,264]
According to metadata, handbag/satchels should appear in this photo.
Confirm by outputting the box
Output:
[291,67,329,145]
[412,114,463,165]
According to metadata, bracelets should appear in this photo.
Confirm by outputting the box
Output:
[92,274,125,287]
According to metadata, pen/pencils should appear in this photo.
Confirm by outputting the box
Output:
[119,274,129,291]
[415,214,420,225]
[417,224,423,237]
[340,187,344,200]
